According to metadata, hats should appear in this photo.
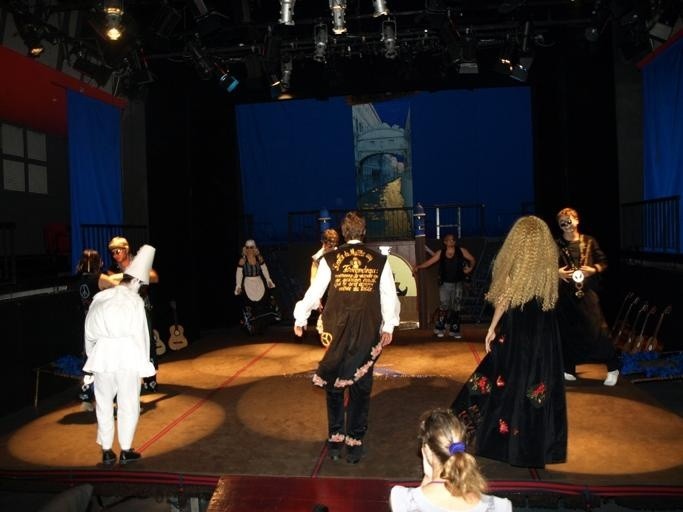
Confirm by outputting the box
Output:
[122,243,157,287]
[108,236,130,250]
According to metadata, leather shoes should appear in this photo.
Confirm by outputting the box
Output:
[118,448,142,466]
[101,448,118,466]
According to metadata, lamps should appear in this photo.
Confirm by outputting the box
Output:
[155,4,239,92]
[260,1,401,106]
[101,2,129,44]
[12,11,50,61]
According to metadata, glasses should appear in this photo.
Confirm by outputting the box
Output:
[111,250,124,256]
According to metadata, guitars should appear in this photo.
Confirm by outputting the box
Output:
[150,307,166,356]
[167,302,188,350]
[606,293,673,355]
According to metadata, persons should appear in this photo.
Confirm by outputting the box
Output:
[449,215,568,469]
[291,209,402,465]
[108,237,160,394]
[412,234,476,339]
[555,207,621,387]
[233,239,275,335]
[80,273,160,465]
[388,407,513,512]
[310,230,339,334]
[65,247,120,412]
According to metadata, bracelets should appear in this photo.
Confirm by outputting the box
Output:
[592,264,597,273]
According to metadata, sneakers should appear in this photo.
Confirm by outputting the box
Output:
[564,371,577,382]
[328,445,363,466]
[603,369,620,387]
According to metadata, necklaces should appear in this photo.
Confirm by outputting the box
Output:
[428,481,448,484]
